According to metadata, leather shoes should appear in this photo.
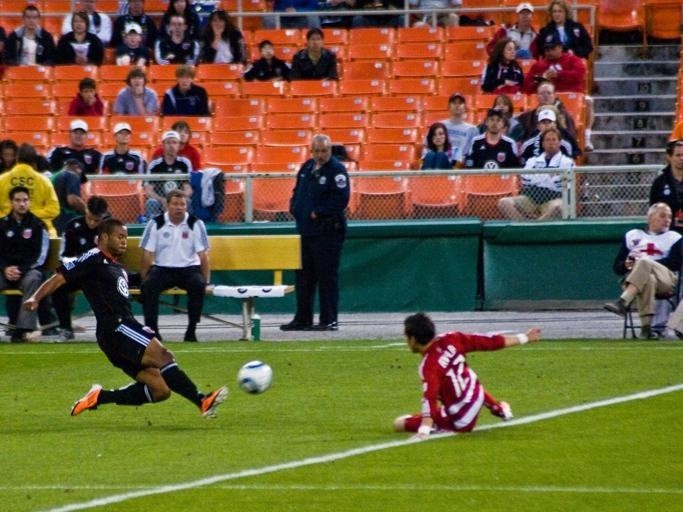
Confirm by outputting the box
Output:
[304,319,338,331]
[280,318,313,332]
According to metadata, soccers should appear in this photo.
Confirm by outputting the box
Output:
[237,361,273,393]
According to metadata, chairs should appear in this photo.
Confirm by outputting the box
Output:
[619,267,682,340]
[593,0,683,53]
[670,53,683,144]
[0,0,594,223]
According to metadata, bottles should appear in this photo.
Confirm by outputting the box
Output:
[249,313,261,340]
[582,177,590,198]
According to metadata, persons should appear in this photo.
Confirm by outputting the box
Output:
[391,310,539,438]
[648,139,682,334]
[354,1,405,28]
[601,201,683,341]
[21,218,231,423]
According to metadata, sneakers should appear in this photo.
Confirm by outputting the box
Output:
[640,321,683,340]
[2,321,78,344]
[70,383,103,419]
[183,329,198,342]
[604,296,630,316]
[500,399,514,422]
[200,384,228,420]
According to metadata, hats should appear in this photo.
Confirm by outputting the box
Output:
[160,130,181,142]
[69,118,88,133]
[487,107,505,119]
[537,107,557,124]
[448,91,466,102]
[112,122,132,135]
[122,21,143,35]
[515,1,535,15]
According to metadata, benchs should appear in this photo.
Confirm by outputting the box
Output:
[0,230,304,349]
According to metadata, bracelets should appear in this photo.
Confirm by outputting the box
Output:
[416,424,430,434]
[516,333,529,344]
